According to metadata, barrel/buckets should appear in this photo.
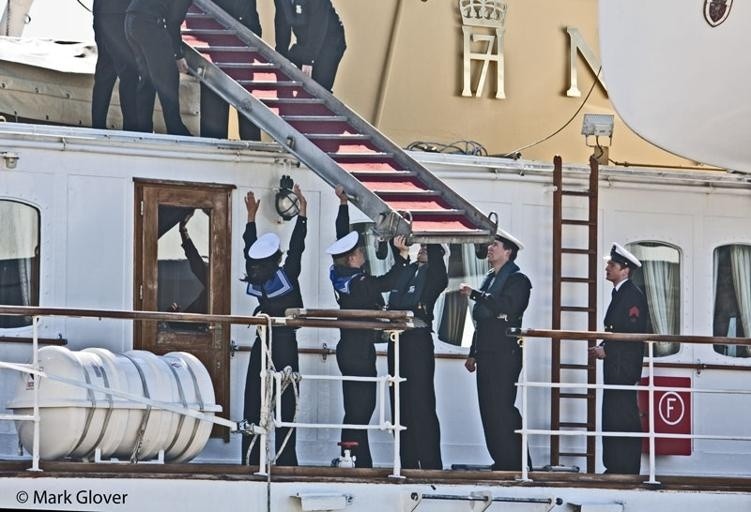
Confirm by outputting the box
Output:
[7,346,216,460]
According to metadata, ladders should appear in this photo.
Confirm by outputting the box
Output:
[550,155,598,474]
[174,0,498,242]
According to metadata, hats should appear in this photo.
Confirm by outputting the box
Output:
[497,228,524,250]
[248,232,281,266]
[439,243,451,257]
[325,230,369,258]
[603,242,642,269]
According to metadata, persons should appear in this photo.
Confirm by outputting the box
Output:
[326,181,412,470]
[165,213,209,333]
[122,0,196,137]
[240,183,311,469]
[585,239,654,475]
[270,0,350,143]
[383,229,452,470]
[185,1,263,143]
[459,227,534,472]
[88,1,142,132]
